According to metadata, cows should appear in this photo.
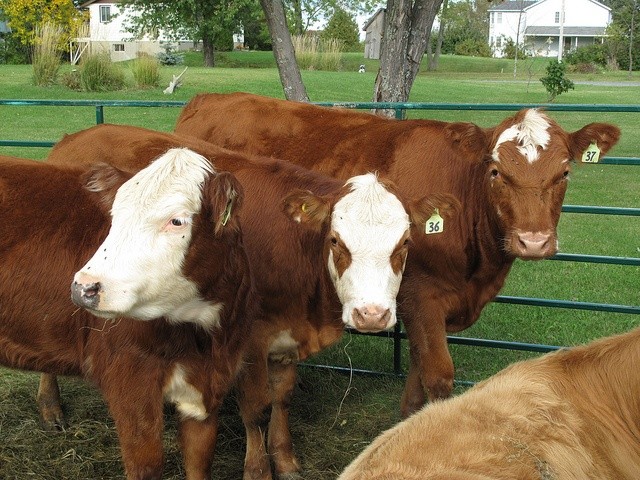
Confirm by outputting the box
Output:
[169,90,622,421]
[35,123,463,480]
[0,133,258,479]
[338,324,640,479]
